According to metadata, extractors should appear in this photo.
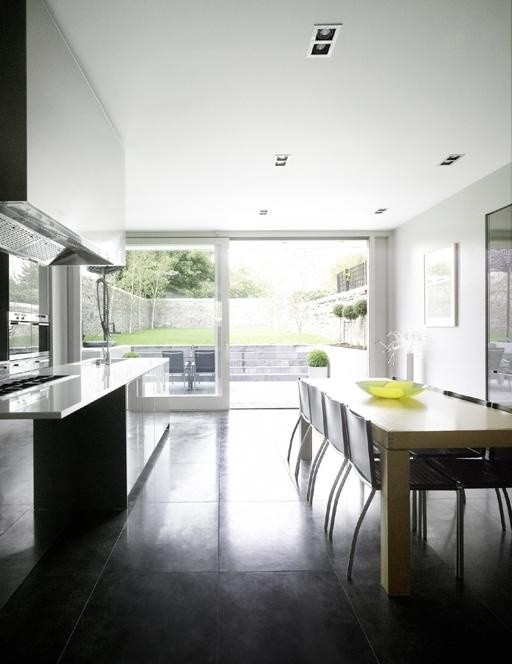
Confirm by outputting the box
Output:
[1,2,128,268]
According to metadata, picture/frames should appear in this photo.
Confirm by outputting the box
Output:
[422,241,458,331]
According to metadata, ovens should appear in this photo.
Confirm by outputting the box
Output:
[6,311,51,360]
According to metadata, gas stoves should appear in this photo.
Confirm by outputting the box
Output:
[0,372,69,399]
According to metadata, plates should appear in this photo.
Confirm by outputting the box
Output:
[357,380,429,399]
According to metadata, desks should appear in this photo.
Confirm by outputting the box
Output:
[300,366,511,601]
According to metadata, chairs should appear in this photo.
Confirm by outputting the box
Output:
[288,375,512,586]
[160,348,217,391]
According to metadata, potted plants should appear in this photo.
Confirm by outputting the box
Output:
[306,348,330,379]
